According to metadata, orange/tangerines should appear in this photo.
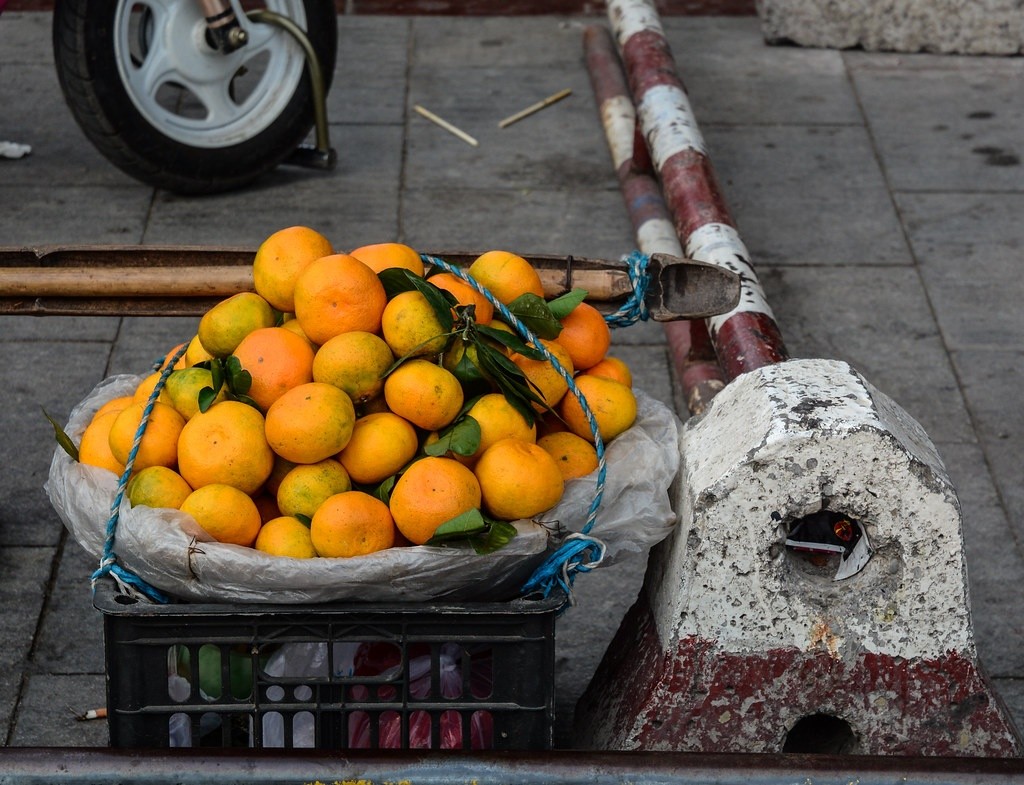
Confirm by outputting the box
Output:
[79,225,639,558]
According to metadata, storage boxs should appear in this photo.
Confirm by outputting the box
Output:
[93,575,569,749]
